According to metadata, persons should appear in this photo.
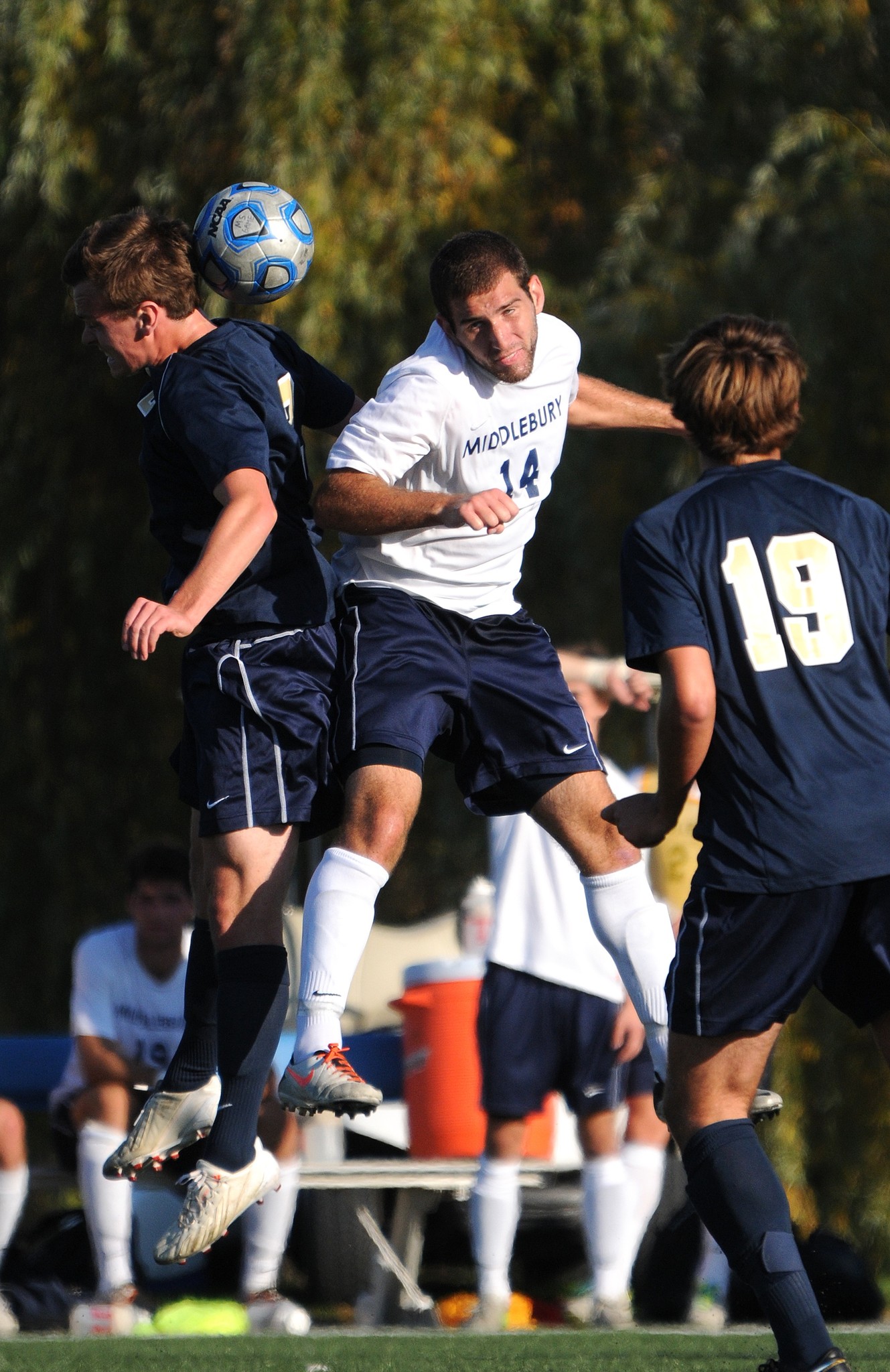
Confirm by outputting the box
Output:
[0,845,725,1339]
[602,319,890,1372]
[275,231,790,1120]
[60,212,364,1267]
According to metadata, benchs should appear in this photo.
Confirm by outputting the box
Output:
[299,1159,584,1310]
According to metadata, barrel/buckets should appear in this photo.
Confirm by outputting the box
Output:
[387,958,554,1167]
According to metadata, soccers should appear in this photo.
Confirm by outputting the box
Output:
[191,178,315,304]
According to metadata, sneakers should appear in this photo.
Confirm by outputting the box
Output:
[276,1044,383,1119]
[151,1135,282,1265]
[104,1072,222,1182]
[650,1071,782,1123]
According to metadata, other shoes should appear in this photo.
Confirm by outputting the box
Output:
[592,1297,634,1330]
[757,1347,853,1372]
[69,1302,136,1335]
[690,1297,725,1331]
[454,1300,509,1337]
[249,1297,311,1336]
[0,1297,19,1340]
